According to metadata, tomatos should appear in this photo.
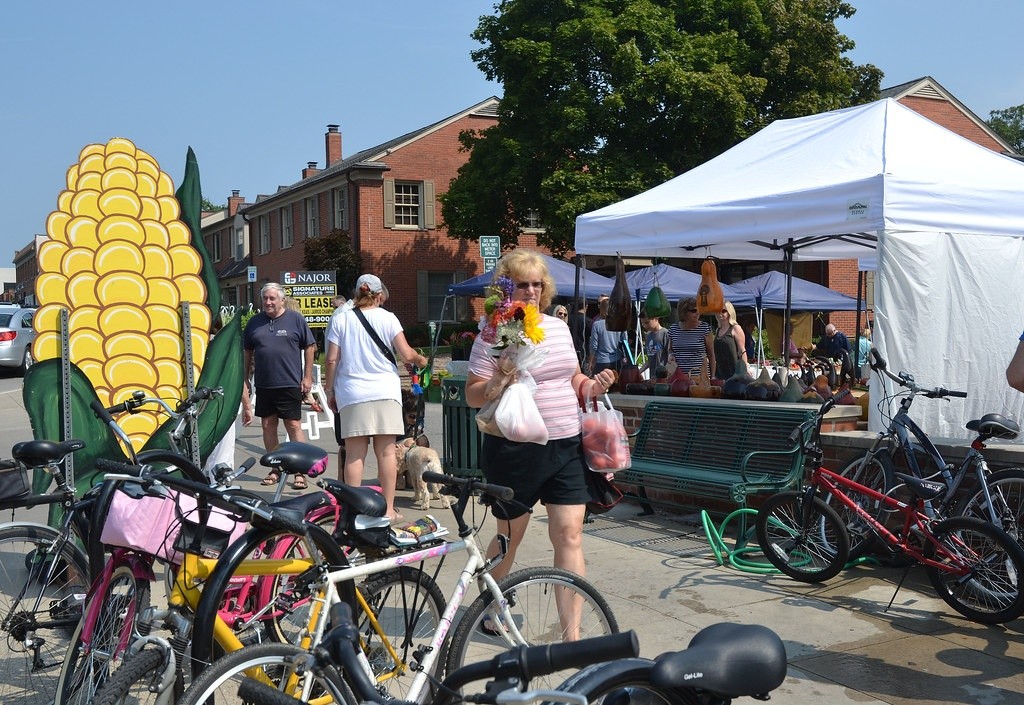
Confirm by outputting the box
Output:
[511,417,547,442]
[581,416,631,470]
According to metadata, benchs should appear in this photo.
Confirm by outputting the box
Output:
[585,397,817,552]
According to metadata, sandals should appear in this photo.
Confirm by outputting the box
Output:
[294,473,307,490]
[260,468,282,484]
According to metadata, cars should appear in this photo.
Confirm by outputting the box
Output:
[0,300,41,377]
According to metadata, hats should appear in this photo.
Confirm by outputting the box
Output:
[355,274,382,294]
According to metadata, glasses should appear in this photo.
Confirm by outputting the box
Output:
[722,309,727,313]
[557,311,567,318]
[511,282,541,289]
[405,412,424,421]
[269,319,274,333]
[687,309,697,314]
[826,330,834,336]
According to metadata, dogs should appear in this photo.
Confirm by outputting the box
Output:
[394,445,450,510]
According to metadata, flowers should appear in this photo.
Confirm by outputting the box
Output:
[483,272,547,355]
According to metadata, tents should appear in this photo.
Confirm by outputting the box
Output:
[855,257,876,368]
[573,96,1024,447]
[435,251,873,360]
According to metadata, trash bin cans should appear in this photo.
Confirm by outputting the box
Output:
[442,375,485,496]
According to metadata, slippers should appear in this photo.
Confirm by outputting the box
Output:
[481,615,506,637]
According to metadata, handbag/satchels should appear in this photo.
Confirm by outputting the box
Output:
[579,377,630,473]
[580,378,623,515]
[496,381,548,446]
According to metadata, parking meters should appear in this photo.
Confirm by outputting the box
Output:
[425,321,437,364]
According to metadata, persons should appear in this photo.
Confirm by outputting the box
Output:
[203,313,252,485]
[326,272,429,526]
[243,283,316,488]
[286,297,300,314]
[551,294,755,382]
[815,324,851,360]
[853,328,873,365]
[779,319,806,362]
[397,389,425,448]
[465,246,614,642]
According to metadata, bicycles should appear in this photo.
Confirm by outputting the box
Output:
[816,347,1023,607]
[0,385,789,703]
[755,386,1023,625]
